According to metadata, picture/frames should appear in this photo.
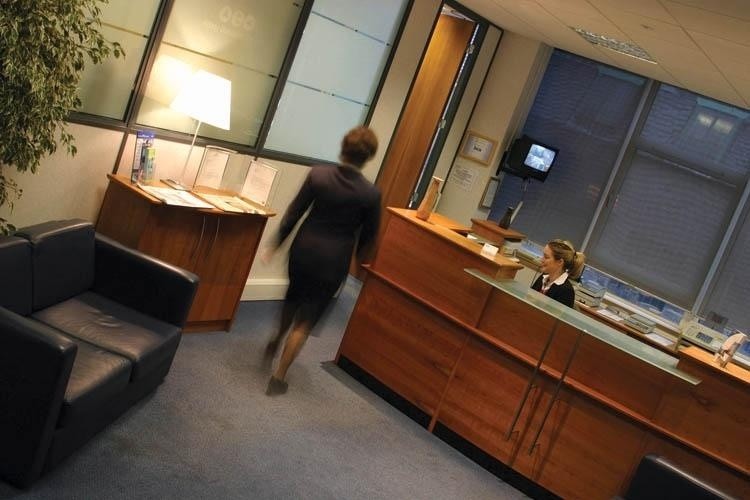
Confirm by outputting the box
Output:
[456,128,499,166]
[479,174,501,210]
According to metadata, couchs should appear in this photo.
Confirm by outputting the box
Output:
[0,218,200,491]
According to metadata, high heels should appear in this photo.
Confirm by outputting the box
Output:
[265,376,290,396]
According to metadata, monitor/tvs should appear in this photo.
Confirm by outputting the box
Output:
[506,134,560,183]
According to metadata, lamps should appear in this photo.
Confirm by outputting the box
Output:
[159,69,232,192]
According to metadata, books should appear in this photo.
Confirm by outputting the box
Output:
[713,327,748,368]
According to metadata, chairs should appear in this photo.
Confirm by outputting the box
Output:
[624,454,733,500]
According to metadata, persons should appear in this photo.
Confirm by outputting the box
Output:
[530,239,586,309]
[259,124,382,397]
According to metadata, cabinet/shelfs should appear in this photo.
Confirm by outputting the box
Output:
[92,172,277,335]
